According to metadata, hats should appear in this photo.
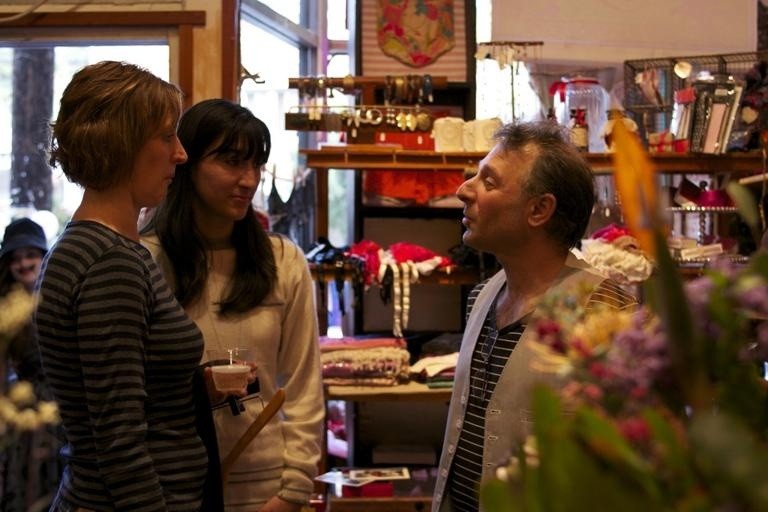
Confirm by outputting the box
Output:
[0,215,48,263]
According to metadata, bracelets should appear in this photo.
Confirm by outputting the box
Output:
[293,72,437,105]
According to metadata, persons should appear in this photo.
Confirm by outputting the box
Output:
[27,56,263,512]
[428,117,648,512]
[135,96,328,512]
[0,214,67,512]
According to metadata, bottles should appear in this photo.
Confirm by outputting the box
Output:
[569,108,589,153]
[552,73,610,154]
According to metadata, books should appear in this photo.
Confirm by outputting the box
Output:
[210,377,262,416]
[370,443,437,467]
[674,243,724,261]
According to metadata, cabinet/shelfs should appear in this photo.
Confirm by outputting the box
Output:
[276,78,488,512]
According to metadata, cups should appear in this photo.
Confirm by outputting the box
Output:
[206,348,252,392]
